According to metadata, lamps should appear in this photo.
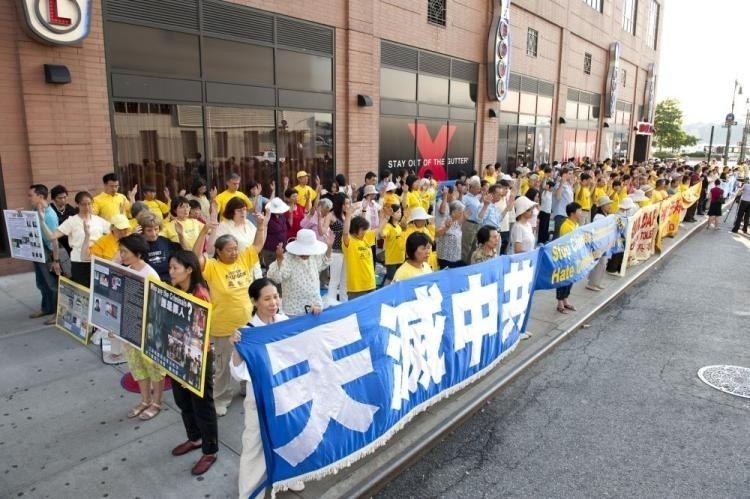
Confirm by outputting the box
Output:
[357,95,374,107]
[604,122,609,127]
[44,63,72,85]
[559,116,566,124]
[633,126,639,130]
[488,108,500,117]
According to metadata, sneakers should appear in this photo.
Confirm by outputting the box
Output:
[705,218,750,235]
[43,314,58,327]
[594,284,606,290]
[585,285,601,291]
[27,308,51,318]
[556,306,570,315]
[563,303,577,312]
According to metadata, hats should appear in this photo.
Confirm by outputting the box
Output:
[596,194,615,210]
[406,206,434,224]
[110,214,132,230]
[296,170,312,178]
[284,227,330,257]
[361,184,381,197]
[618,197,635,210]
[385,181,398,192]
[629,189,650,203]
[264,196,292,215]
[512,196,540,220]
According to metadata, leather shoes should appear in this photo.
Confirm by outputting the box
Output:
[190,452,218,477]
[171,437,202,459]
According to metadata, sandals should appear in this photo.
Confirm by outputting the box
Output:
[126,400,153,419]
[138,401,163,423]
